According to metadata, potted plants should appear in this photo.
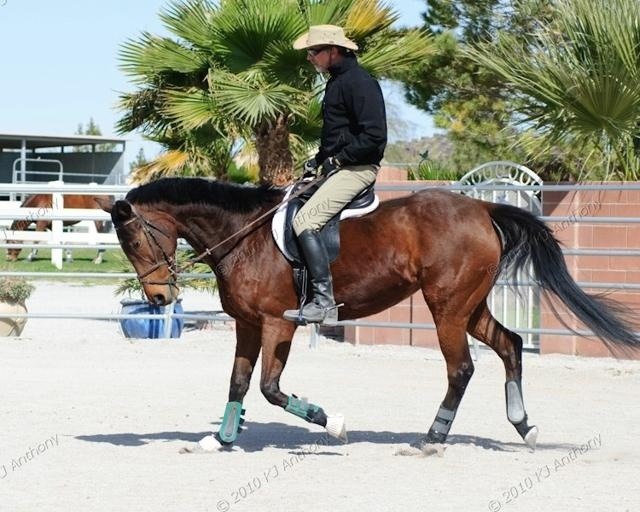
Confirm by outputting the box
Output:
[0,275,36,338]
[107,249,201,338]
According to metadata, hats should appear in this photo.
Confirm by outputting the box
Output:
[292,24,359,51]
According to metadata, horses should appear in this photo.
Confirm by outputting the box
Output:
[6,194,116,265]
[93,176,640,456]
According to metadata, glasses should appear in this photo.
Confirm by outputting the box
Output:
[307,45,338,57]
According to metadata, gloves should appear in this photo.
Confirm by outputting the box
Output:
[310,156,342,181]
[303,158,318,175]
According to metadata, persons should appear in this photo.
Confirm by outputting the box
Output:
[282,25,388,328]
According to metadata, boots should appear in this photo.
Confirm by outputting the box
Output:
[281,227,339,327]
